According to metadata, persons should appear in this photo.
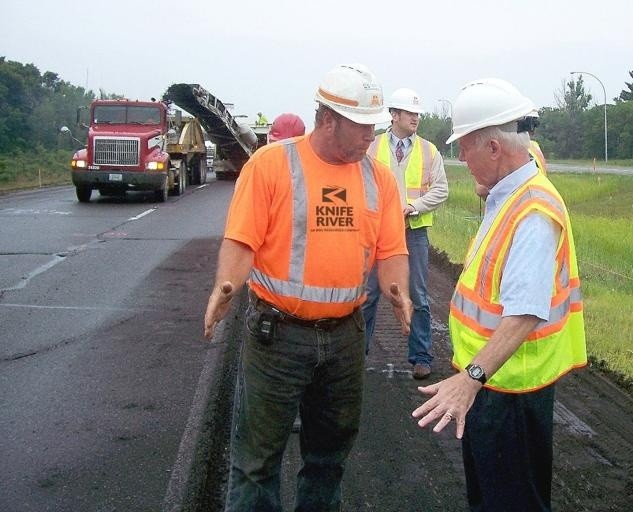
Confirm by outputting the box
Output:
[407,79,588,512]
[475,111,548,201]
[361,88,449,380]
[257,112,268,127]
[205,62,413,512]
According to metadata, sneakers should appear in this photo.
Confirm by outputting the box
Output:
[413,362,430,378]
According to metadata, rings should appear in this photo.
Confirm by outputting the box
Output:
[444,412,454,420]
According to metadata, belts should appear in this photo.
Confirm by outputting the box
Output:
[257,301,338,331]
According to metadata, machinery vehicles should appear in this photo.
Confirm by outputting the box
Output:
[59,98,210,204]
[163,84,268,180]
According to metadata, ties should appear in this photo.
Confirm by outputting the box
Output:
[396,142,404,161]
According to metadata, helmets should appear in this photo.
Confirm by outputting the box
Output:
[446,79,540,144]
[313,63,394,124]
[387,87,423,115]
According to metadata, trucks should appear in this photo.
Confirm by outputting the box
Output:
[204,145,216,171]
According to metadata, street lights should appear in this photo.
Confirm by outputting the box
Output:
[438,98,453,158]
[569,71,608,165]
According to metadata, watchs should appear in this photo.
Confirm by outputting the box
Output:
[464,364,487,385]
